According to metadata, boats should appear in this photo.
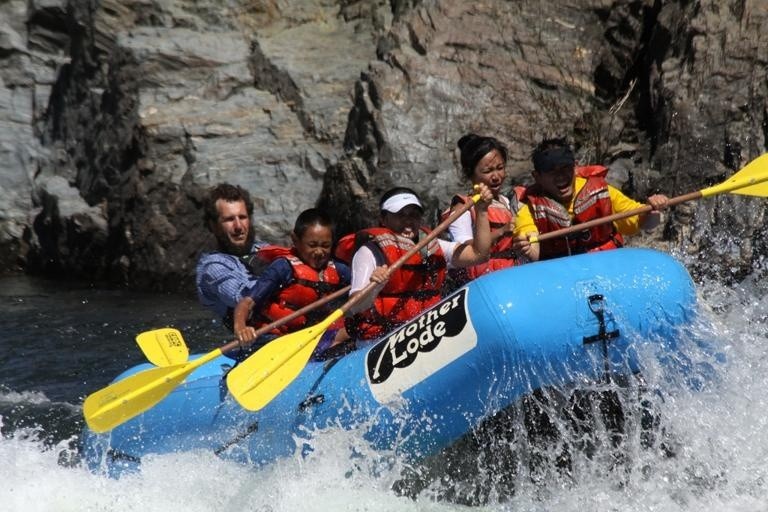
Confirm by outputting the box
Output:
[63,246,728,481]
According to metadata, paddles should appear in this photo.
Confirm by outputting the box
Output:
[135,327,189,366]
[83,284,350,434]
[226,185,489,411]
[528,153,768,243]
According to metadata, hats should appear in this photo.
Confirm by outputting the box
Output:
[534,147,575,170]
[380,193,423,214]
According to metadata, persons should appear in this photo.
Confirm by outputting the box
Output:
[445,131,525,281]
[233,207,351,361]
[508,136,671,263]
[348,181,494,350]
[193,181,274,341]
